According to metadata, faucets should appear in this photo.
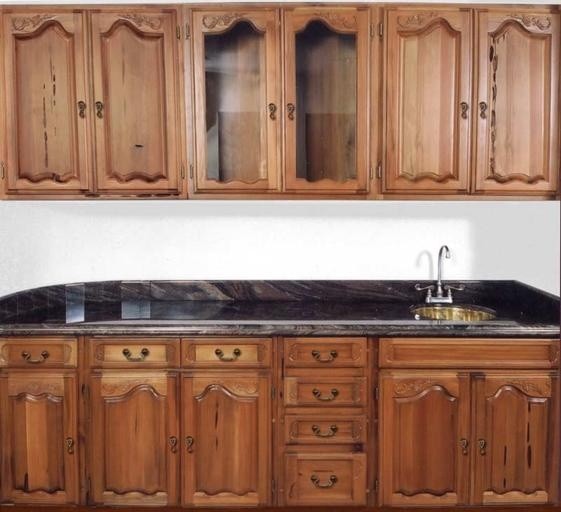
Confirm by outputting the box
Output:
[435,246,450,308]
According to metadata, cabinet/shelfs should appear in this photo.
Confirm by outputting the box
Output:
[2,336,82,509]
[84,335,276,512]
[1,4,186,201]
[187,6,381,201]
[377,335,559,511]
[278,336,376,511]
[381,5,560,202]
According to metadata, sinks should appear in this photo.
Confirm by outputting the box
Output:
[410,303,497,322]
